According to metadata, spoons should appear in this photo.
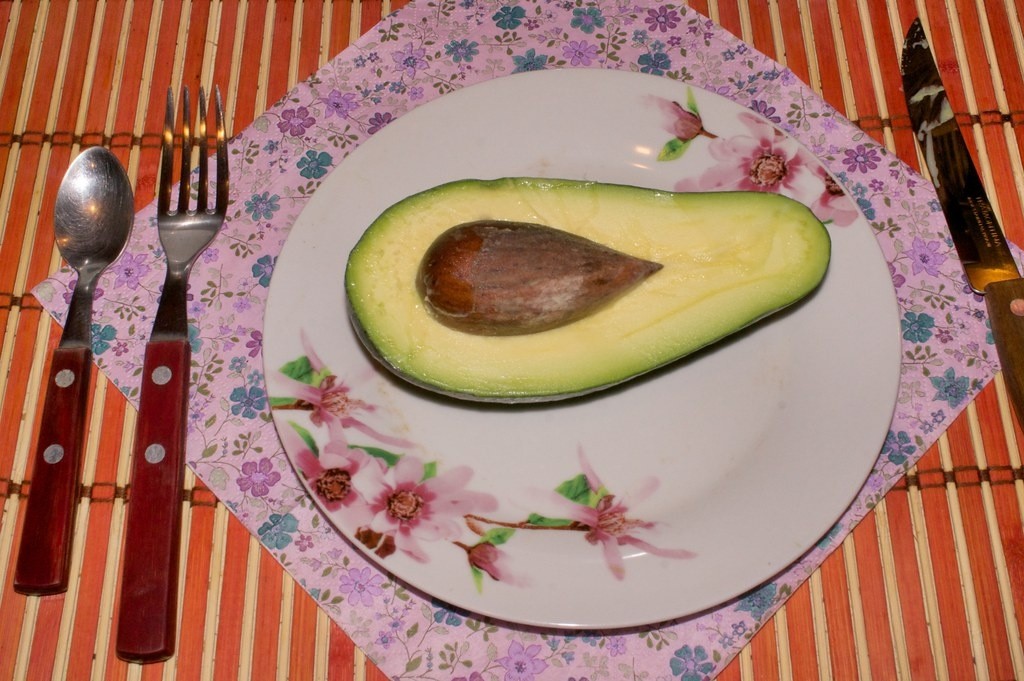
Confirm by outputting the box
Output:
[8,144,137,599]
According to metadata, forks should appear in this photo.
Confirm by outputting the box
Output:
[112,86,231,666]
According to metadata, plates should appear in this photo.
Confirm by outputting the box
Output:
[258,65,908,633]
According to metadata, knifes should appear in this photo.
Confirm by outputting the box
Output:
[902,15,1023,445]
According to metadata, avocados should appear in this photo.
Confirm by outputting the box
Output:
[343,177,831,406]
